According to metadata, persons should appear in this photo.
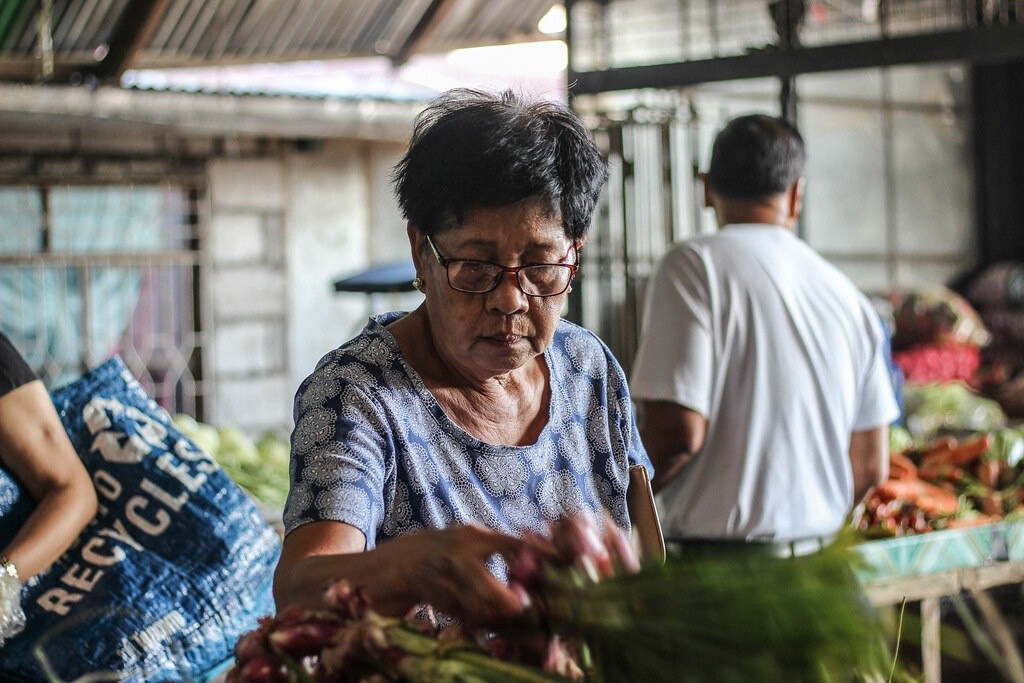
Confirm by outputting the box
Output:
[0,329,98,643]
[892,290,1023,415]
[272,95,660,623]
[624,117,899,569]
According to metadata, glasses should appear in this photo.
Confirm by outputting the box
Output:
[427,233,579,298]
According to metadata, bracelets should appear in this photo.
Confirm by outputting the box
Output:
[0,557,21,583]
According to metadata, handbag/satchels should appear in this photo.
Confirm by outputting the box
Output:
[0,355,287,682]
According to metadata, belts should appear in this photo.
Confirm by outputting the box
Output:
[666,537,840,559]
